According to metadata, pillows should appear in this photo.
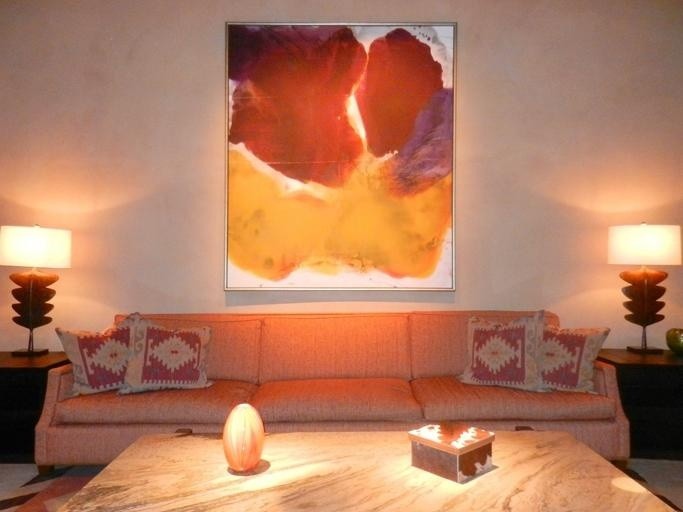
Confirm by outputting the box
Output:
[115,313,216,398]
[539,324,610,395]
[55,312,144,398]
[456,311,552,393]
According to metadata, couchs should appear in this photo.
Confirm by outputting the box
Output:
[33,310,631,476]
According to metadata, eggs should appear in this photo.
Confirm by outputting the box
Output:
[221,402,264,472]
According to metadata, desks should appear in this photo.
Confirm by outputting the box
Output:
[55,430,680,512]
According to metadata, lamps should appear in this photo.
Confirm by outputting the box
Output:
[0,224,72,359]
[607,221,682,354]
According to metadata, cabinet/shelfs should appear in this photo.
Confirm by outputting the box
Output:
[0,352,70,464]
[597,349,683,461]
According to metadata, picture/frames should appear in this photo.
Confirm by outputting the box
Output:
[223,20,458,291]
